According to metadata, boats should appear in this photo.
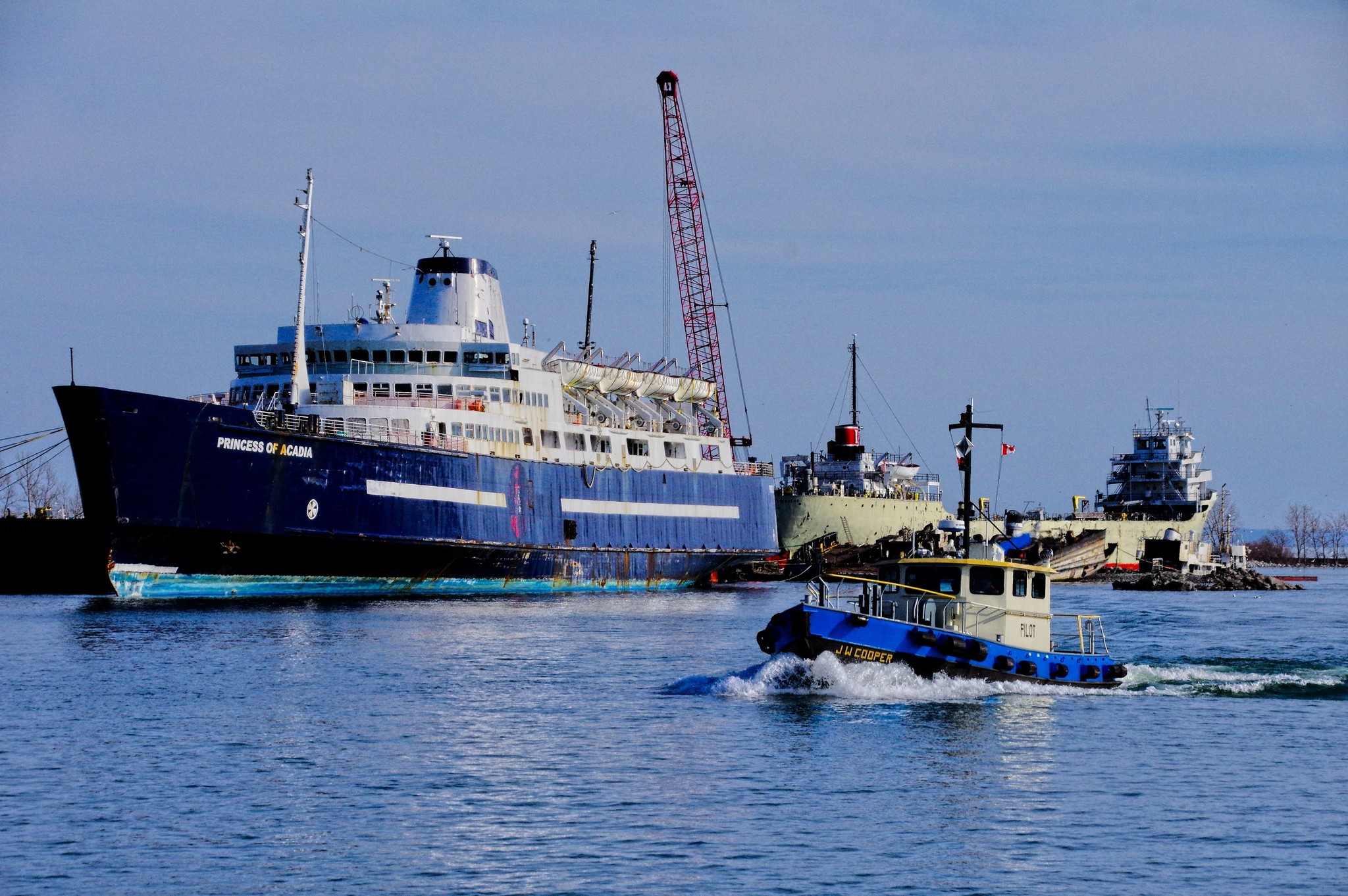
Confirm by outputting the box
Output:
[1015,527,1119,582]
[758,403,1125,690]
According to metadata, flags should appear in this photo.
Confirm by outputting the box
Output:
[1001,443,1016,455]
[955,447,965,465]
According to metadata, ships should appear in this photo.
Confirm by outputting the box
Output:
[46,160,766,611]
[770,332,955,557]
[934,396,1249,576]
